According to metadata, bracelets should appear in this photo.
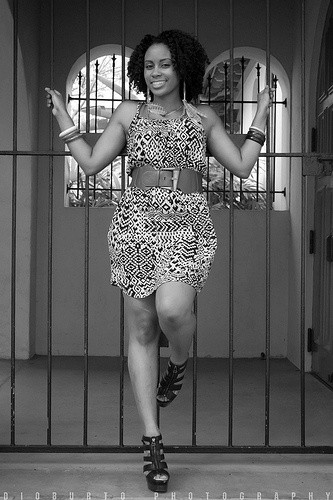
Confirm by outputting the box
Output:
[59,126,83,143]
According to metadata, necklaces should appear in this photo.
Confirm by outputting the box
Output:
[147,103,184,116]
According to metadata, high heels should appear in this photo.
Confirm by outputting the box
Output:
[141,433,169,493]
[156,358,188,407]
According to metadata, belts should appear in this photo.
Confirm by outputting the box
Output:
[130,165,203,193]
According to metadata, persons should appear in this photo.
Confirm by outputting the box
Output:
[44,30,274,492]
[246,126,265,146]
